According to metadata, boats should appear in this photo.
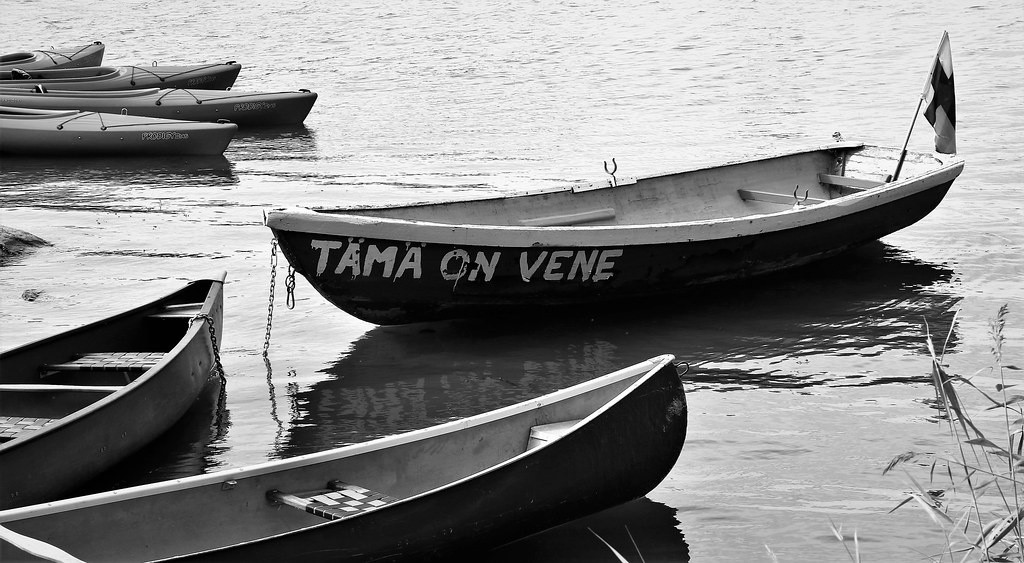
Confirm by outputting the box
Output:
[0,103,238,158]
[255,22,965,327]
[0,39,107,70]
[0,350,690,563]
[0,59,243,90]
[0,83,320,128]
[1,268,232,503]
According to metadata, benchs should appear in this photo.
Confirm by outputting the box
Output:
[46,352,168,382]
[271,481,403,519]
[0,416,60,440]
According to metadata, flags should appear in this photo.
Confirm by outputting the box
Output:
[920,31,956,156]
